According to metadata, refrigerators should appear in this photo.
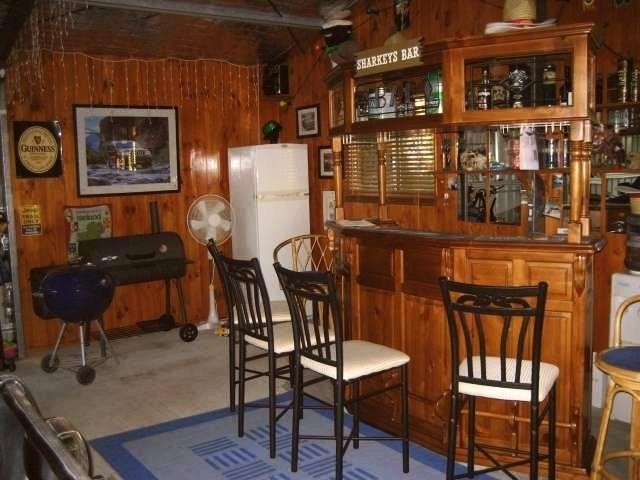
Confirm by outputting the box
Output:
[224,140,316,326]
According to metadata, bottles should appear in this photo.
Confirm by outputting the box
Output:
[542,137,559,169]
[476,60,492,113]
[541,63,557,105]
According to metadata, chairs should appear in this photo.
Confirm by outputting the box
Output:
[39,263,122,384]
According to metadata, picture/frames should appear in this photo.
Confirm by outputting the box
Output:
[295,103,319,140]
[71,102,182,197]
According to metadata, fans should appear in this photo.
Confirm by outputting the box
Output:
[185,192,237,331]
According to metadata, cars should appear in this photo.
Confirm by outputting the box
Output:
[100,140,152,167]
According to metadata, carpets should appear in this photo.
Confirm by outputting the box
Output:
[87,388,491,479]
